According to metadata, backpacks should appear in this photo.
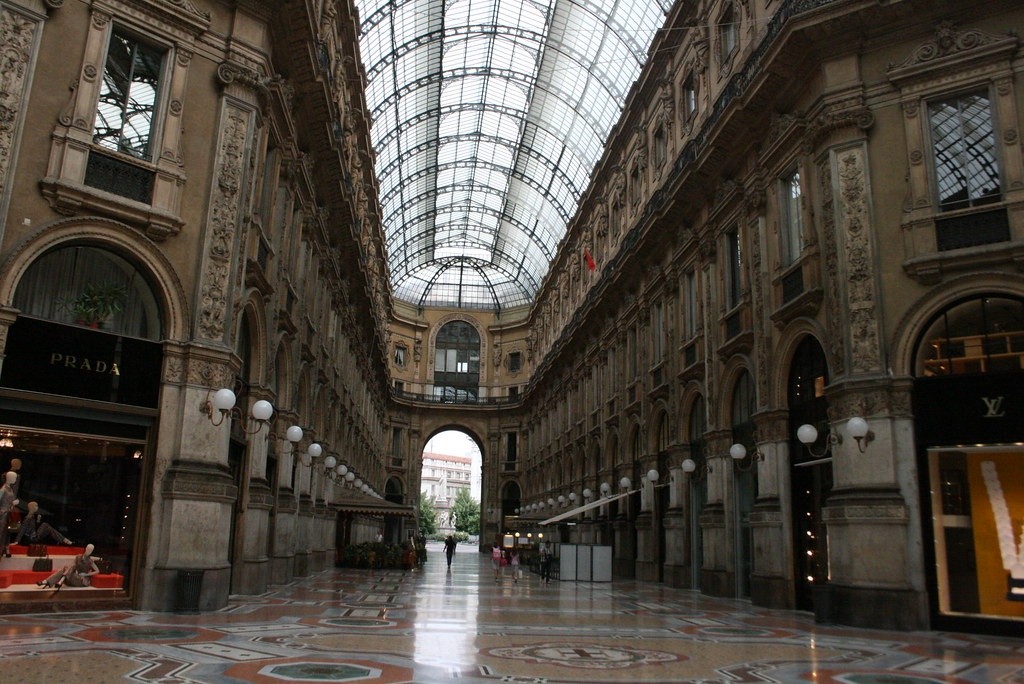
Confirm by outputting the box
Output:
[511,553,519,565]
[492,546,501,560]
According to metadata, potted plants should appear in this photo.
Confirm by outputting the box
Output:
[483,541,560,579]
[341,539,419,572]
[54,279,131,331]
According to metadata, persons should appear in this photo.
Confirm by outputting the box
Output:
[0,458,22,557]
[10,502,78,547]
[36,543,99,591]
[375,530,385,547]
[0,471,21,557]
[490,541,522,582]
[538,540,554,584]
[443,534,458,568]
[415,532,426,570]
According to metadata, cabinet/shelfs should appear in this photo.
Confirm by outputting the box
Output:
[922,330,1024,376]
[1,543,125,596]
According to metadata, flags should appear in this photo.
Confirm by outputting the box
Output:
[584,247,596,272]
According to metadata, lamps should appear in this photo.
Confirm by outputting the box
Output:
[198,387,236,427]
[795,423,844,459]
[514,532,520,545]
[647,469,674,493]
[620,478,645,497]
[845,417,877,454]
[514,482,622,516]
[526,532,532,545]
[0,437,15,448]
[681,458,713,485]
[306,456,384,501]
[290,443,322,467]
[730,443,762,474]
[538,532,543,543]
[264,426,304,457]
[226,398,274,435]
